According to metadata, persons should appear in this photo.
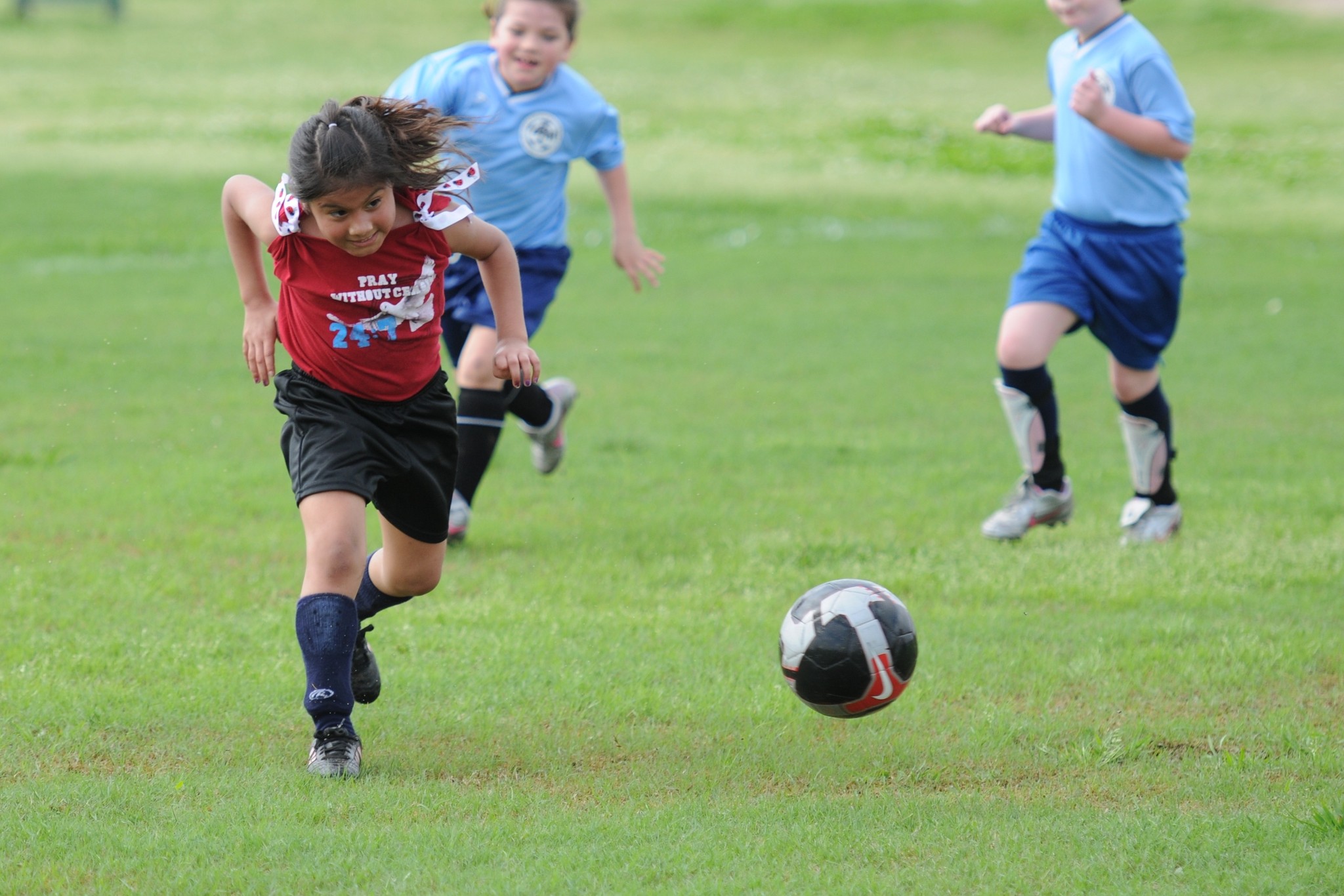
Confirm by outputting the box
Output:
[222,95,544,778]
[971,0,1195,549]
[380,0,665,546]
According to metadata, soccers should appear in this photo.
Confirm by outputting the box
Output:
[772,578,918,722]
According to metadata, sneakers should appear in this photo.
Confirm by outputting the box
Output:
[1117,493,1183,557]
[347,619,382,707]
[517,376,579,476]
[983,472,1071,547]
[304,724,362,783]
[442,484,472,542]
